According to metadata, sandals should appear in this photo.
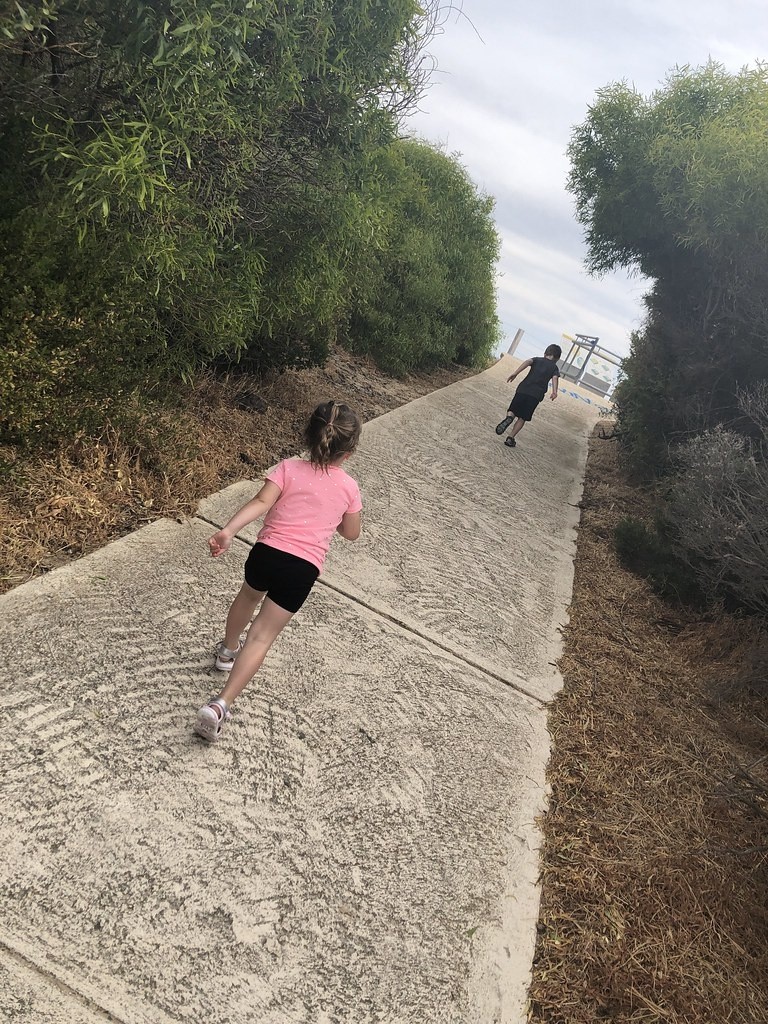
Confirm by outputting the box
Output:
[504,436,518,447]
[495,416,512,434]
[193,696,226,741]
[213,639,242,671]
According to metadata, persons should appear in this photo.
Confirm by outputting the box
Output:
[495,343,562,449]
[193,400,363,743]
